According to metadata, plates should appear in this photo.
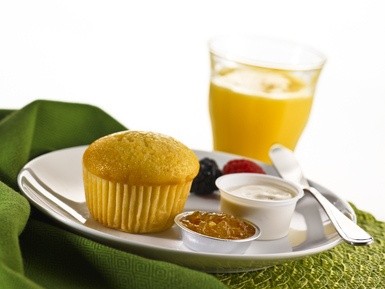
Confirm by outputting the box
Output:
[16,148,358,275]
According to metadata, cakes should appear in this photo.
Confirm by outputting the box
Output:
[83,130,201,235]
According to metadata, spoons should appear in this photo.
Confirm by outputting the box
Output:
[268,142,374,246]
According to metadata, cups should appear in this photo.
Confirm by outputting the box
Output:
[206,37,328,167]
[173,210,261,255]
[215,172,304,241]
